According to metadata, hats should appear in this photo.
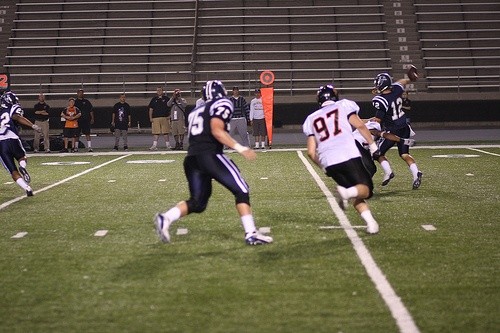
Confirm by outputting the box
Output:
[255,88,260,93]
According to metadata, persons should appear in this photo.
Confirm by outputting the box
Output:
[224,86,253,150]
[401,90,417,147]
[148,86,172,151]
[167,88,187,150]
[0,89,42,196]
[249,88,267,149]
[190,85,211,112]
[302,84,384,234]
[74,89,94,152]
[152,80,273,245]
[59,97,81,153]
[34,94,52,153]
[112,94,131,151]
[352,65,422,190]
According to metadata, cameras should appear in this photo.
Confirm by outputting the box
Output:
[176,92,180,97]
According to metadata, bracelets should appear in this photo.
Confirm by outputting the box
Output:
[128,121,131,123]
[234,143,249,153]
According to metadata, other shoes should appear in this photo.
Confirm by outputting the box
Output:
[33,147,93,153]
[148,145,158,151]
[254,141,260,149]
[175,142,183,150]
[26,185,33,196]
[165,146,173,151]
[19,166,30,183]
[113,146,118,151]
[124,145,128,151]
[335,185,348,210]
[365,224,379,235]
[261,142,265,149]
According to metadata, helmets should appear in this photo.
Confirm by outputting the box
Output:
[373,71,393,93]
[316,83,338,109]
[202,80,227,103]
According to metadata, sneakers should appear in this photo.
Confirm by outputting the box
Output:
[244,231,274,246]
[381,171,395,186]
[412,170,423,190]
[154,212,170,243]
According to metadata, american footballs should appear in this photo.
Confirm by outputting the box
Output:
[408,65,419,81]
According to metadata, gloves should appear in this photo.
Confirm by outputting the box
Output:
[400,138,416,147]
[251,121,254,126]
[368,143,380,160]
[322,167,327,176]
[32,125,42,133]
[247,119,250,126]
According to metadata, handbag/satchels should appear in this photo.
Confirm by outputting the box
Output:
[184,110,189,127]
